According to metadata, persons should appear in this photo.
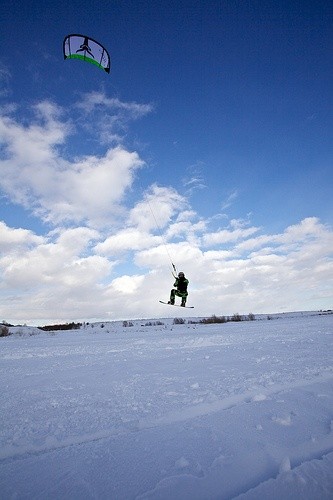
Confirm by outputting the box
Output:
[168,271,189,306]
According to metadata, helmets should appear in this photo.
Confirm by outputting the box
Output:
[178,272,184,278]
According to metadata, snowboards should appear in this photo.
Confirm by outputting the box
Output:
[159,300,195,308]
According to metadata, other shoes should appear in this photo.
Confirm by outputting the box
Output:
[168,300,174,304]
[181,303,185,307]
